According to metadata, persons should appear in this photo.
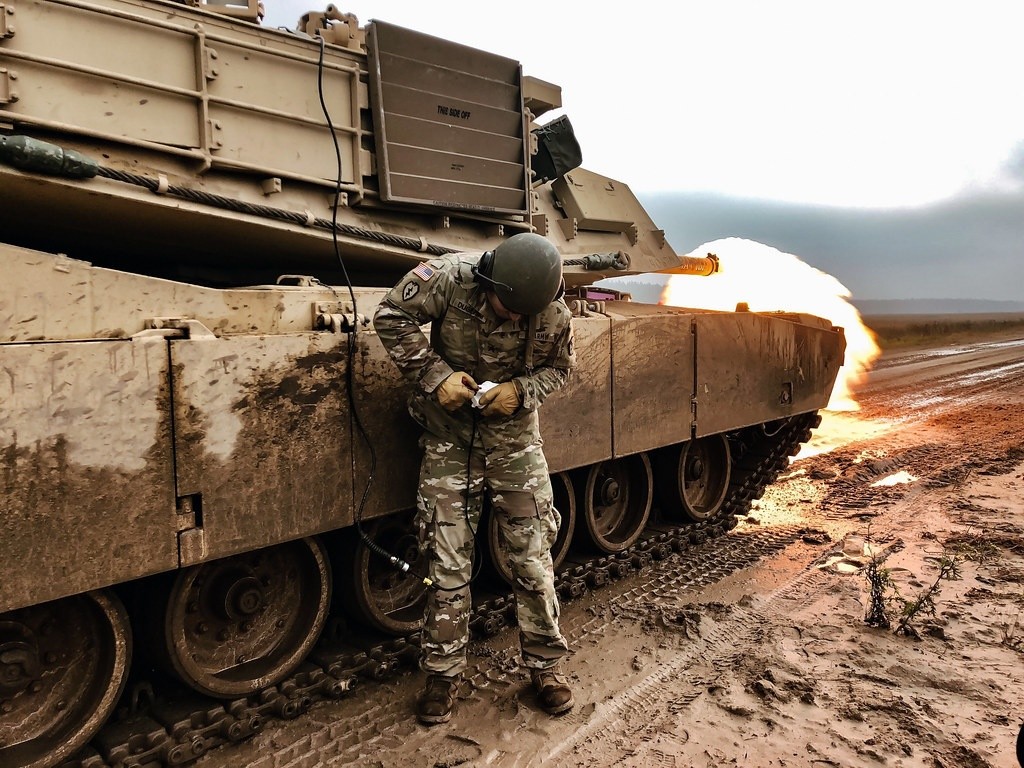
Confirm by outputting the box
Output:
[372,232,579,724]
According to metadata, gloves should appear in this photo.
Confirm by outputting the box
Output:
[437,371,478,411]
[478,380,522,420]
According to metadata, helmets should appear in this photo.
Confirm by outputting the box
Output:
[491,233,562,316]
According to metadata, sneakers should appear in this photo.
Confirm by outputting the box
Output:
[417,675,462,724]
[531,662,574,714]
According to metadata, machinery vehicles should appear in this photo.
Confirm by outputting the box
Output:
[0,0,845,768]
